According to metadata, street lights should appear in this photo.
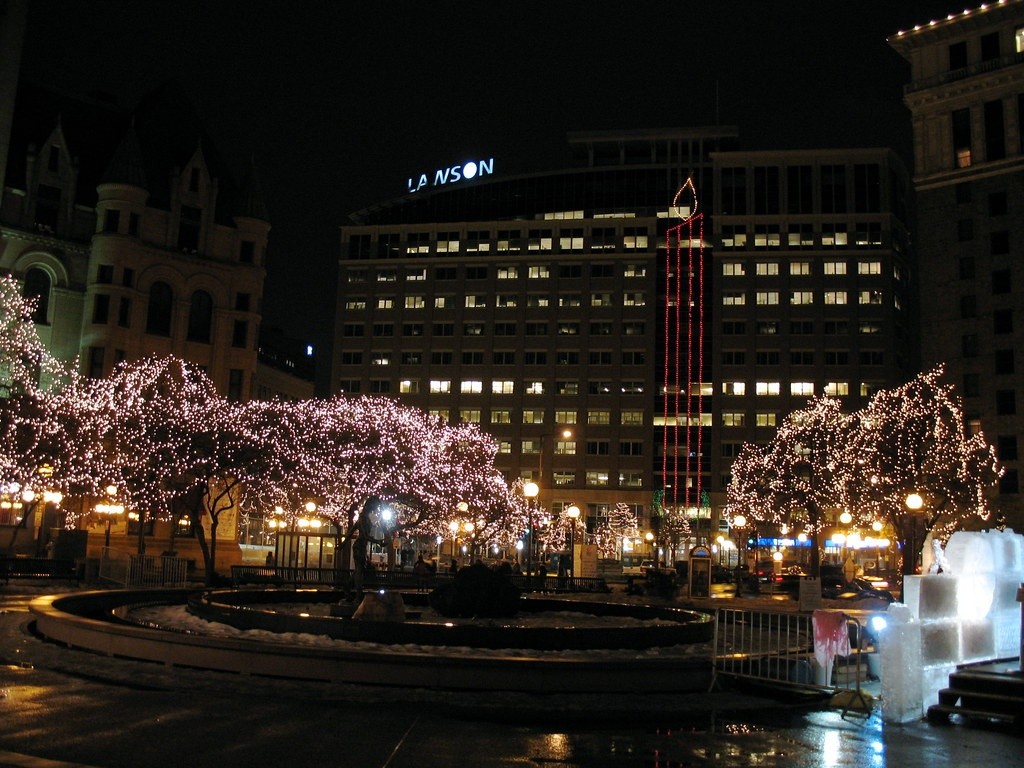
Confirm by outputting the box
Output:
[95,485,125,547]
[523,482,540,587]
[839,512,853,578]
[567,505,581,587]
[734,515,747,599]
[906,494,924,575]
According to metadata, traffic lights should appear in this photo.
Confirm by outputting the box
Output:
[746,534,758,550]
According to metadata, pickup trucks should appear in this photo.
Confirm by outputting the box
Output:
[621,559,677,586]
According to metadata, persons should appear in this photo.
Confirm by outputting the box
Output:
[45,539,90,578]
[265,551,274,566]
[400,545,547,591]
